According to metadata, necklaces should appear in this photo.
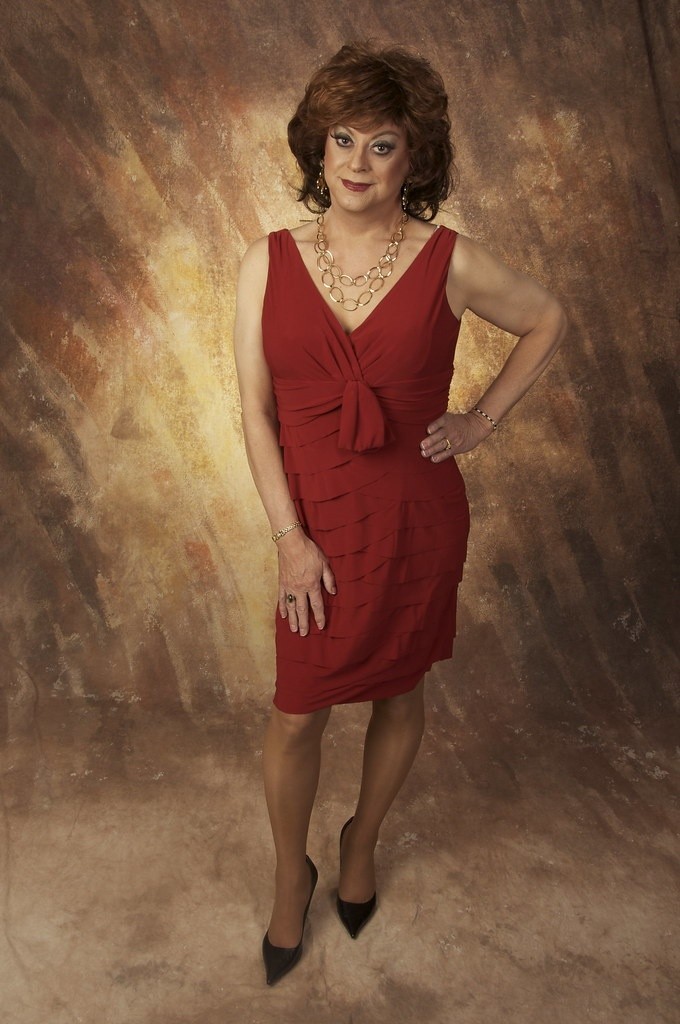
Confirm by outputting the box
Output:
[314,209,410,312]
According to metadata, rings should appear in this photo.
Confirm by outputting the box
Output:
[286,594,296,603]
[445,438,451,450]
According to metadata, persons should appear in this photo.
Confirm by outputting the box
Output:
[233,37,569,984]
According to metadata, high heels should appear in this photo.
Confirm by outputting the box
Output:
[262,854,318,986]
[336,816,377,939]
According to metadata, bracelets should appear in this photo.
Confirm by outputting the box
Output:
[272,520,303,542]
[472,406,497,432]
